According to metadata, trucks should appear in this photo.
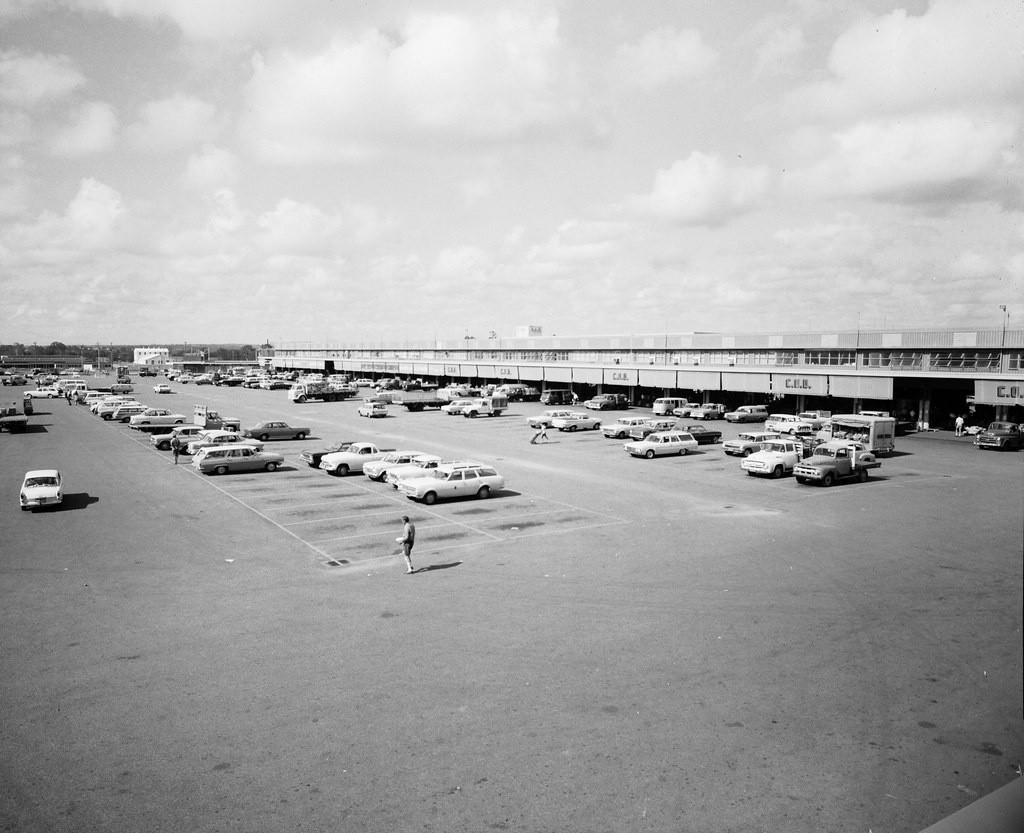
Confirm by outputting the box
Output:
[740,438,827,479]
[791,443,883,487]
[811,413,896,458]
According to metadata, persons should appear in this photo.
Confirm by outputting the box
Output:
[538,422,550,443]
[570,390,579,406]
[65,389,79,405]
[955,414,964,437]
[170,435,180,465]
[36,379,41,388]
[396,516,415,574]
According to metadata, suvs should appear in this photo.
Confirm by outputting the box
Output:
[362,449,425,482]
[689,402,726,421]
[724,405,768,423]
[673,402,700,419]
[721,431,782,457]
[397,460,505,505]
[763,413,815,437]
[191,445,286,475]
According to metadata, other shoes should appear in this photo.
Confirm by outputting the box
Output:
[406,570,413,574]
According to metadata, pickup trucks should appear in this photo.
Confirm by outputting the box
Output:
[300,439,397,470]
[797,412,831,430]
[318,441,403,476]
[385,452,480,489]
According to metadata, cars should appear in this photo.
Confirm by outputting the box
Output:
[973,421,1021,452]
[599,417,652,439]
[623,431,698,459]
[19,469,64,512]
[0,365,628,468]
[669,423,722,444]
[629,419,676,442]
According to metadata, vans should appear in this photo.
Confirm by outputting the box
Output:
[651,397,688,417]
